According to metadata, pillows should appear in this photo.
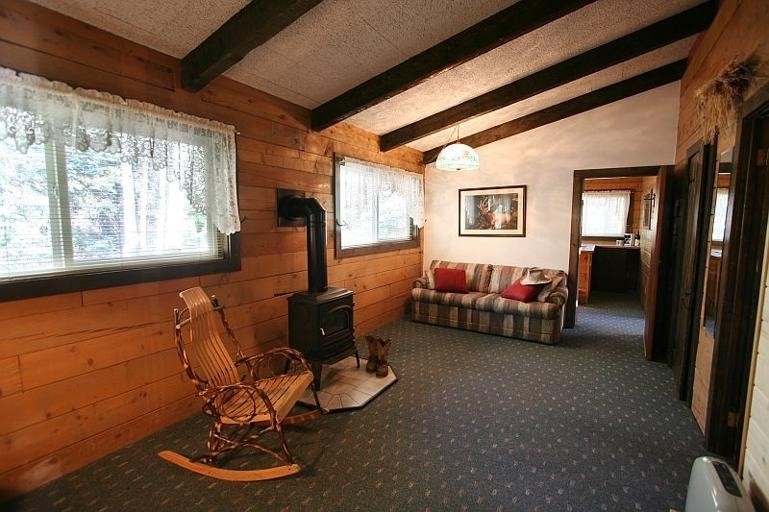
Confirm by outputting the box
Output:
[424,267,469,293]
[500,276,562,302]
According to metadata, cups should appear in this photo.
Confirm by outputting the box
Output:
[616,239,624,247]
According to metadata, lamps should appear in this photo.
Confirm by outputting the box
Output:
[435,124,480,171]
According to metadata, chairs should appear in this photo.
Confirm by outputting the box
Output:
[157,287,329,482]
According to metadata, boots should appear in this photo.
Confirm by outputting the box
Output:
[375,338,392,377]
[366,336,377,372]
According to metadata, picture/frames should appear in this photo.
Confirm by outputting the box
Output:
[459,186,526,236]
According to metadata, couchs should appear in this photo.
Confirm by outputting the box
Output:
[410,260,569,345]
